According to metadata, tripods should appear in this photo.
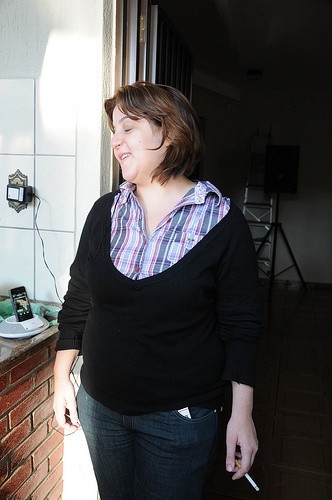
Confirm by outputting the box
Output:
[255,197,307,319]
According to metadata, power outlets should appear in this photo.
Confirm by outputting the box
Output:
[8,169,27,212]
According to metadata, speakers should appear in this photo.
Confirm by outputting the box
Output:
[263,144,301,193]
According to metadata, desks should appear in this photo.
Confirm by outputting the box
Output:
[0,295,65,500]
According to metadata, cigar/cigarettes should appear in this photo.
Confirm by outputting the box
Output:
[234,459,260,491]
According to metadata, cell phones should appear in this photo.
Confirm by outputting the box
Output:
[8,286,34,322]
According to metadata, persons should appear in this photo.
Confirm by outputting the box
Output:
[54,82,268,500]
